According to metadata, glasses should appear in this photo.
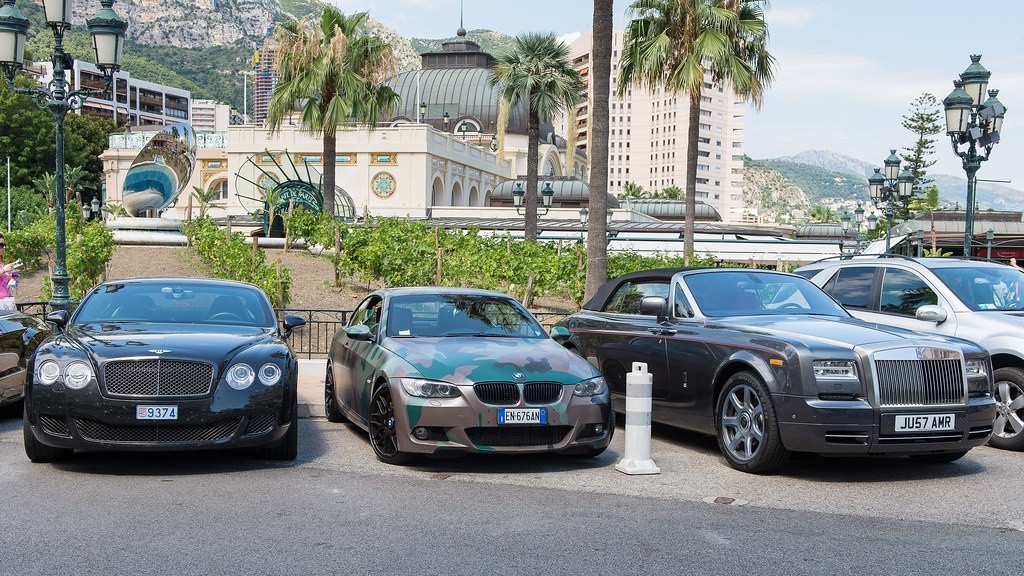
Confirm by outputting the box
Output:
[0,243,6,248]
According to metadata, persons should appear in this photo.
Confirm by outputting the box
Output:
[0,232,18,312]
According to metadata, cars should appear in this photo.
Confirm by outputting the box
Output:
[552,267,997,474]
[23,276,306,462]
[325,286,616,466]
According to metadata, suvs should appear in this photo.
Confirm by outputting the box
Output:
[791,252,1024,452]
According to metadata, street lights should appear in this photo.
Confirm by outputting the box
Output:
[838,241,844,260]
[492,136,496,152]
[986,228,995,259]
[868,149,915,258]
[917,227,924,257]
[478,128,483,146]
[579,206,614,228]
[461,121,466,140]
[512,183,554,218]
[942,54,1007,259]
[82,203,91,222]
[90,197,100,217]
[420,102,427,123]
[841,204,878,256]
[1,0,130,333]
[288,106,294,125]
[444,112,450,132]
